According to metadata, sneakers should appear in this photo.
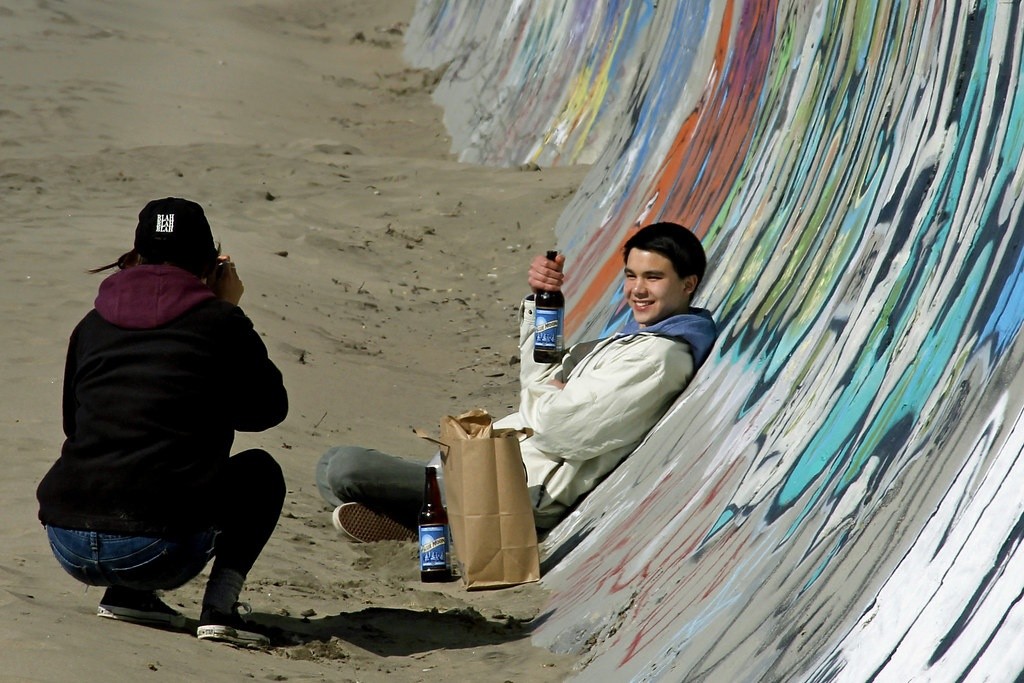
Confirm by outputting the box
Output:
[197,604,272,646]
[96,585,186,629]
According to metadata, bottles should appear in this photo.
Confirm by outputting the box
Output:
[419,467,452,583]
[534,251,564,363]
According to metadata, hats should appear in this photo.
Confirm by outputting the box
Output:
[134,197,217,278]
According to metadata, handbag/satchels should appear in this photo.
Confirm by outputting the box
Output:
[409,408,540,591]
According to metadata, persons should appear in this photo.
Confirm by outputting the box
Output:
[315,221,718,542]
[36,197,289,645]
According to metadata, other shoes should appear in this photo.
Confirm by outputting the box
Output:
[333,501,419,543]
